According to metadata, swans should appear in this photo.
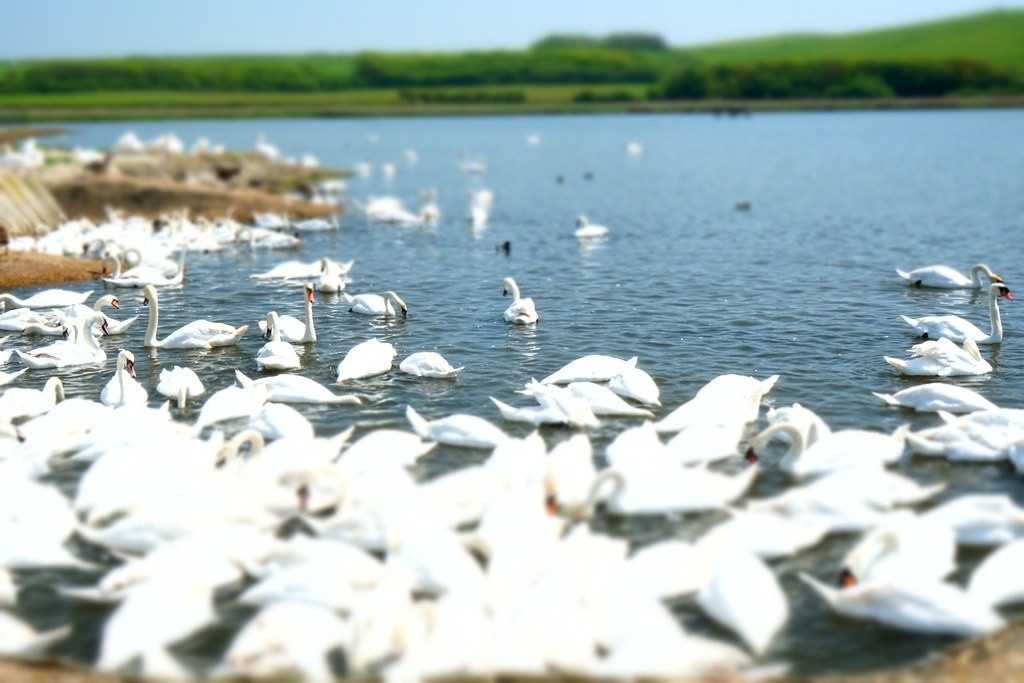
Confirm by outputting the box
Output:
[0,130,1024,683]
